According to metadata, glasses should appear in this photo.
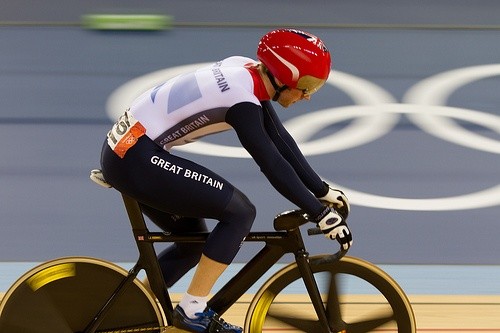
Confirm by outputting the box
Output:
[303,88,317,96]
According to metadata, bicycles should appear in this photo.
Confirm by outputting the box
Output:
[0,167,417,333]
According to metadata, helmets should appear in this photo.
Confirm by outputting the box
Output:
[256,28,331,91]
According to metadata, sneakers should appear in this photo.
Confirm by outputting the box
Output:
[170,302,244,333]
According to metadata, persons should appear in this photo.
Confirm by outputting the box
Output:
[100,28,352,333]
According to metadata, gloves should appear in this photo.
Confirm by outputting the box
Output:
[315,185,350,220]
[316,207,354,250]
